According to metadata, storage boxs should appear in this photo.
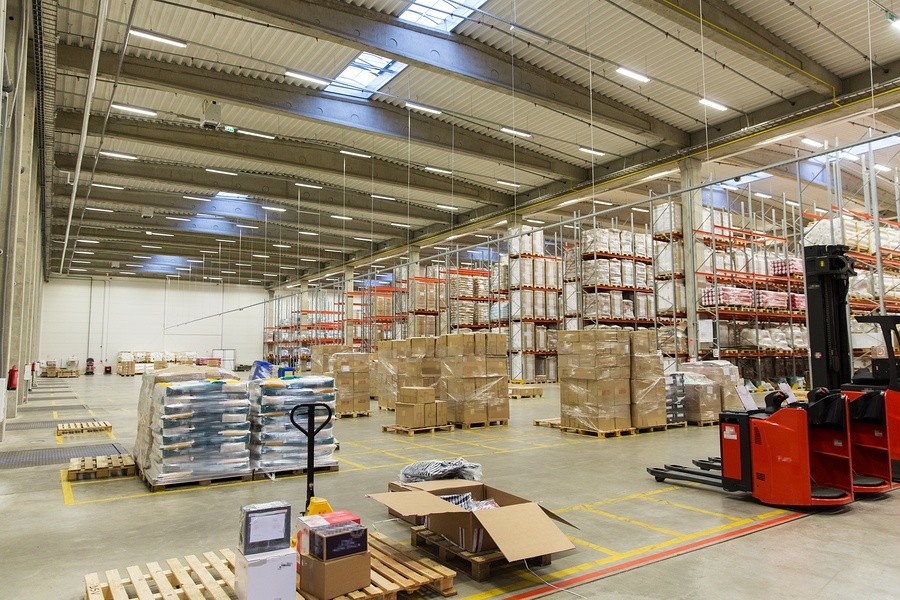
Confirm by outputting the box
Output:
[297,510,371,600]
[297,326,743,434]
[365,478,582,563]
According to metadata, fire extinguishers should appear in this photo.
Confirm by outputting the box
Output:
[32,363,35,372]
[6,364,18,391]
